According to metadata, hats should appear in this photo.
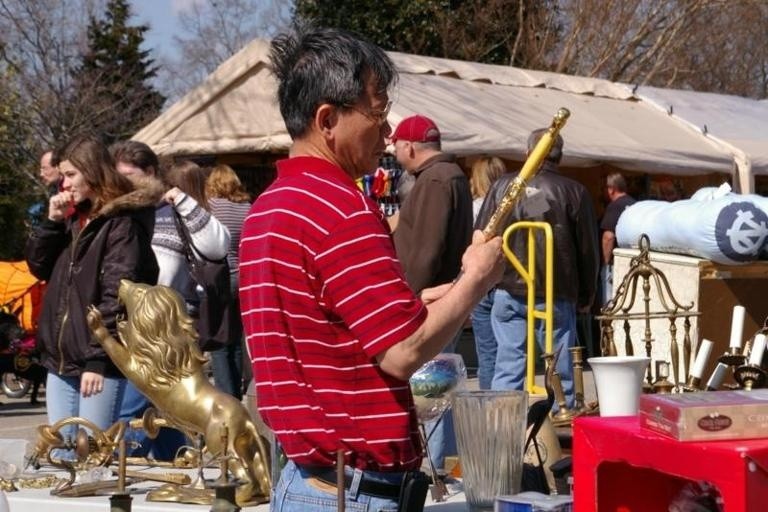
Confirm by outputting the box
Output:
[389,114,440,143]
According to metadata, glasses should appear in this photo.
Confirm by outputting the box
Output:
[340,101,393,126]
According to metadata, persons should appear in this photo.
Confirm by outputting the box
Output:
[388,114,475,474]
[238,24,506,511]
[471,129,599,412]
[25,136,252,462]
[600,173,636,305]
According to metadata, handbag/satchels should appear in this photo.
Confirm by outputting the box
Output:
[171,205,235,352]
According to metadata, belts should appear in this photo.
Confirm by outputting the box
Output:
[308,466,400,501]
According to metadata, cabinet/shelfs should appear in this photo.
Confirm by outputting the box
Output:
[606,245,767,392]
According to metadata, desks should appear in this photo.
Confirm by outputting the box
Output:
[570,412,768,512]
[2,432,473,511]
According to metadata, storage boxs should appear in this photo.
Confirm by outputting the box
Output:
[639,392,768,443]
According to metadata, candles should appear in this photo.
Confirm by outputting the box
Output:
[729,303,747,349]
[748,331,768,366]
[707,361,730,388]
[688,338,715,378]
